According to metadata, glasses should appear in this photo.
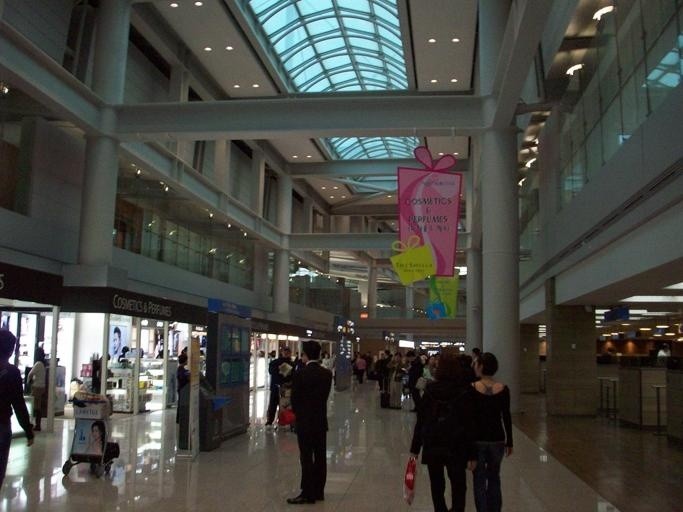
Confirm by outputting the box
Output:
[300,351,305,357]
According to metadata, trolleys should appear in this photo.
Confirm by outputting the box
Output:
[62,399,120,477]
[276,384,294,432]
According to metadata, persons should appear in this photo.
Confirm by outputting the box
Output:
[182,346,187,354]
[286,339,333,505]
[616,351,623,361]
[257,349,265,357]
[0,329,36,490]
[176,354,190,424]
[155,343,163,359]
[86,421,105,455]
[27,347,46,432]
[113,326,122,352]
[657,342,672,366]
[200,350,205,356]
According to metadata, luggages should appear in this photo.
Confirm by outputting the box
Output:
[378,378,402,409]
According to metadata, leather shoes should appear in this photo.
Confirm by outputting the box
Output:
[286,492,314,504]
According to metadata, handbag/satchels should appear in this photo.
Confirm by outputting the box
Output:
[403,458,418,505]
